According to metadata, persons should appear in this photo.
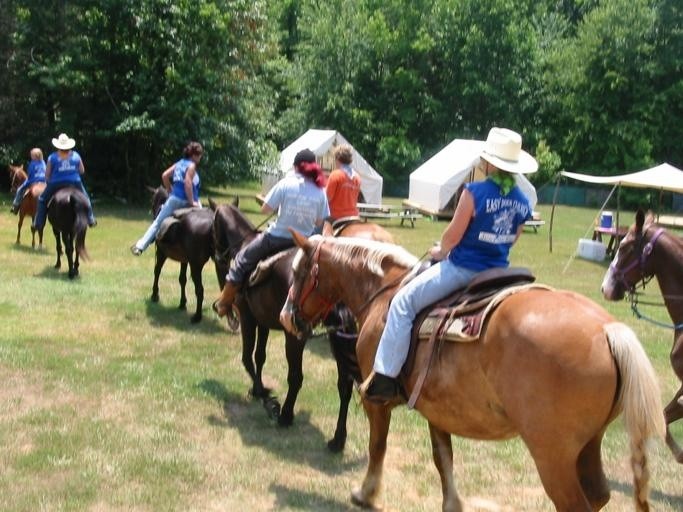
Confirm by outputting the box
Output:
[357,127,540,401]
[130,141,203,256]
[212,149,331,318]
[31,133,97,233]
[9,148,46,216]
[324,145,360,222]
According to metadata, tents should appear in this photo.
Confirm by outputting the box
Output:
[408,138,538,221]
[277,129,383,214]
[550,163,683,253]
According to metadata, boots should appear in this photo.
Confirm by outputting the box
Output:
[10,206,20,215]
[213,282,239,317]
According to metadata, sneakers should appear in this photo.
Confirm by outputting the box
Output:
[366,375,395,401]
[90,221,97,228]
[130,244,142,256]
[33,223,41,230]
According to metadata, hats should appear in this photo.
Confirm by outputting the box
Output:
[51,132,76,150]
[293,148,315,166]
[475,127,539,174]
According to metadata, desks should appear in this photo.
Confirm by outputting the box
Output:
[595,227,627,256]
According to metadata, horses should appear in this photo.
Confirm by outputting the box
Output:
[12,166,49,251]
[600,208,683,462]
[279,222,667,508]
[48,188,91,275]
[205,197,354,451]
[331,218,396,245]
[149,187,221,323]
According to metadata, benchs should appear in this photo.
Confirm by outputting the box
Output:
[356,203,423,228]
[524,220,545,233]
